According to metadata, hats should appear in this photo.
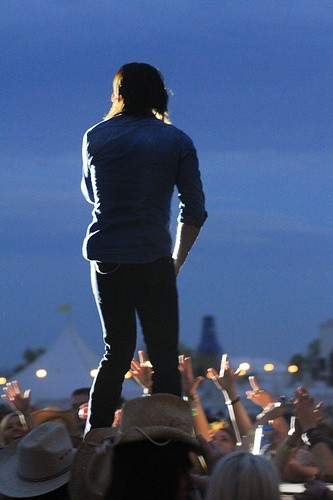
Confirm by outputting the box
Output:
[0,422,79,497]
[84,395,216,457]
[29,406,79,432]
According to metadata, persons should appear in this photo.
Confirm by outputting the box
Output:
[0,352,333,500]
[80,62,207,435]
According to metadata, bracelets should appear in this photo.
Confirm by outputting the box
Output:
[225,397,241,405]
[187,394,199,415]
